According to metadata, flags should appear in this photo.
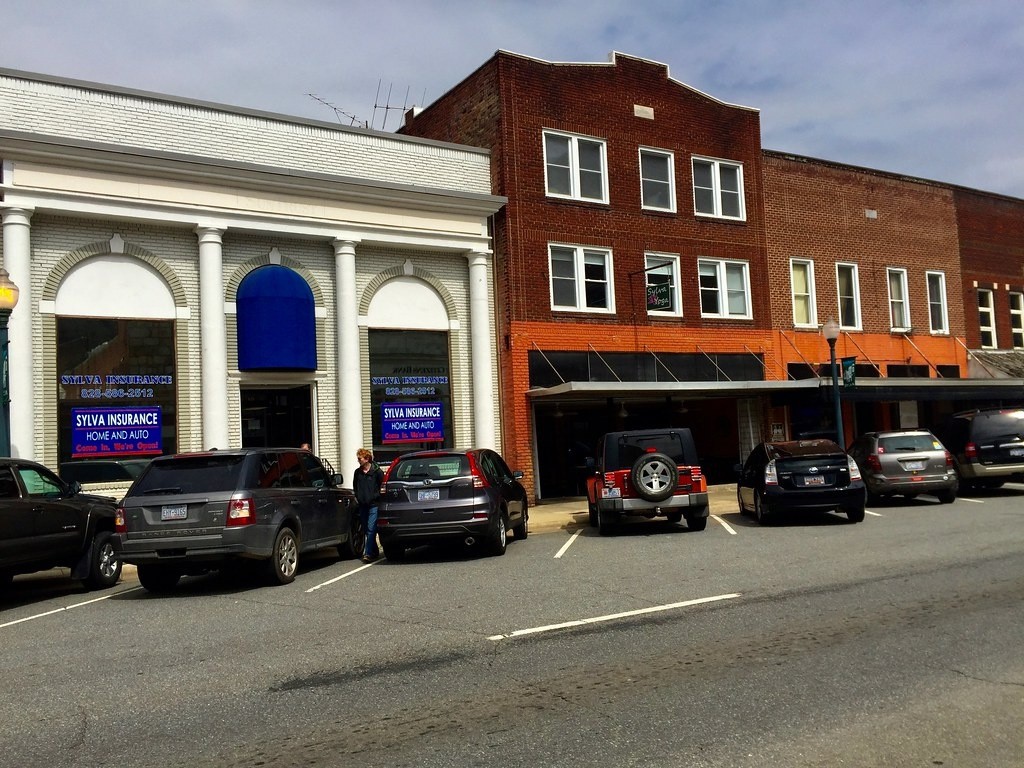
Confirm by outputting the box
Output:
[841,357,856,393]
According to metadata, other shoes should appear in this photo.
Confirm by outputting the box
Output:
[362,556,379,564]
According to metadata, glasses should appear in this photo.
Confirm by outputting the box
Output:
[358,458,361,461]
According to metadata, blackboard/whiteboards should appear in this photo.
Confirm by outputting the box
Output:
[645,282,671,312]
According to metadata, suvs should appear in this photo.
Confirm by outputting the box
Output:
[0,456,122,607]
[114,445,368,594]
[584,427,710,536]
[942,404,1024,495]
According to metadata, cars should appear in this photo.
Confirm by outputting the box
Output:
[735,436,869,526]
[376,446,529,562]
[849,426,959,506]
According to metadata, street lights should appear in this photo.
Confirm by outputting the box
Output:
[823,315,847,453]
[0,265,20,459]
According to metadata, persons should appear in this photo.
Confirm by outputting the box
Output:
[352,448,384,564]
[301,444,312,452]
